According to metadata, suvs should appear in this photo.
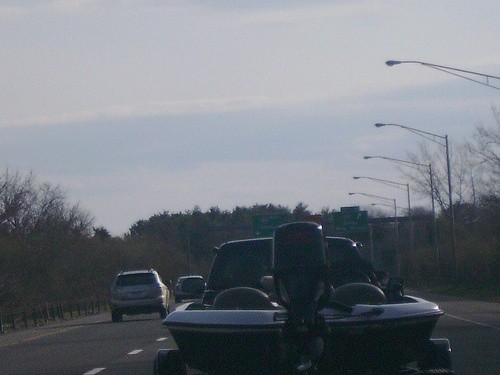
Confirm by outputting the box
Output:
[174,275,209,303]
[109,268,170,322]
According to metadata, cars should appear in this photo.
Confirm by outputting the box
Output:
[150,221,454,375]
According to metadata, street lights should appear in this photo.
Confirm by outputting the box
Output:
[387,60,500,95]
[375,122,462,298]
[354,176,416,293]
[348,192,403,278]
[365,155,441,293]
